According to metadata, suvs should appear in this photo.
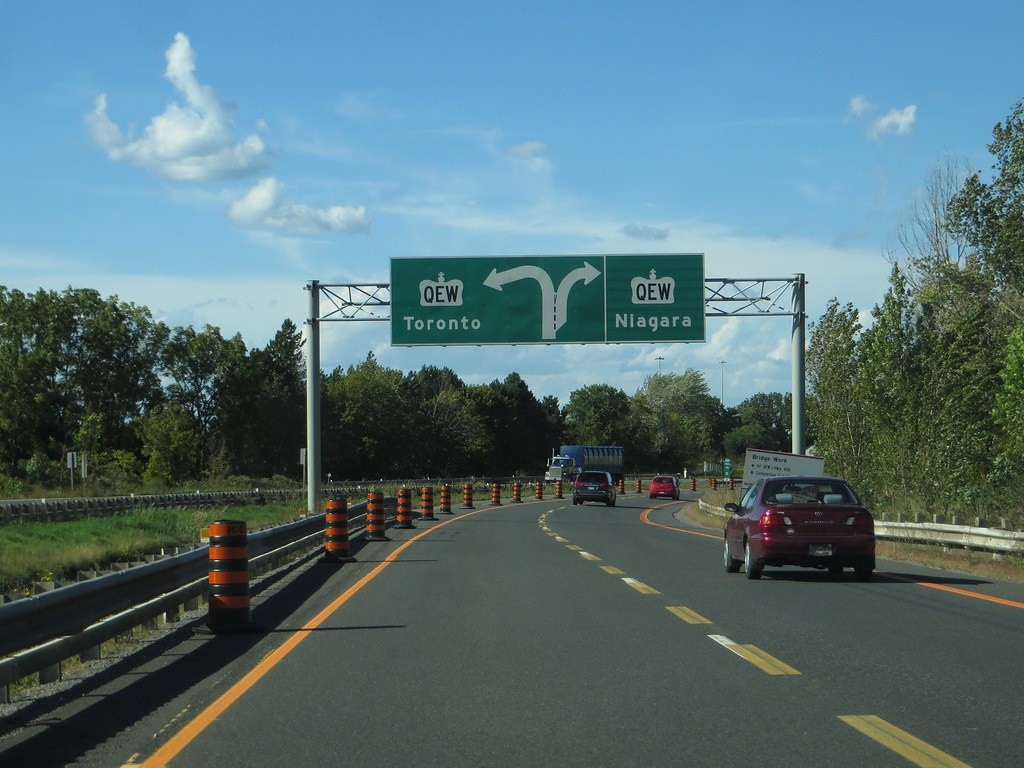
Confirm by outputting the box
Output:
[571,471,618,507]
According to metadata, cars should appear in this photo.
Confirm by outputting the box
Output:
[722,474,876,581]
[649,475,681,500]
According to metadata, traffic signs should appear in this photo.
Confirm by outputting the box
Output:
[387,253,705,349]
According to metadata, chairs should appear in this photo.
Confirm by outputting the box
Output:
[824,495,846,504]
[774,494,795,504]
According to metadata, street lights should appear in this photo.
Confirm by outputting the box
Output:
[718,360,727,403]
[655,356,665,376]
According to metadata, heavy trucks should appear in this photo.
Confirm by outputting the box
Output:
[544,445,625,488]
